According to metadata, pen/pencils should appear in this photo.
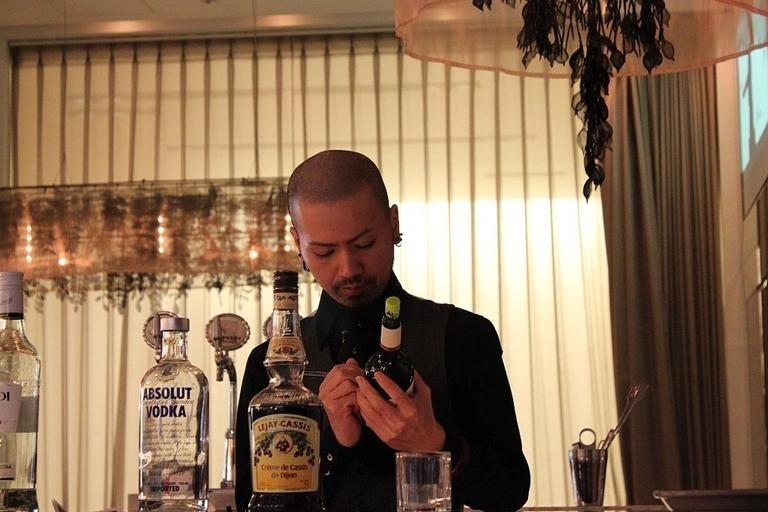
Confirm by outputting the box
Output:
[303,370,329,377]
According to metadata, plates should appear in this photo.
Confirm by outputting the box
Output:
[655,487,767,509]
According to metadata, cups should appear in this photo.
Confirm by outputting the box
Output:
[566,444,611,510]
[394,450,455,511]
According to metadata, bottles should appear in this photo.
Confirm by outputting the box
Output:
[137,314,211,510]
[249,266,327,510]
[0,271,40,512]
[357,297,411,409]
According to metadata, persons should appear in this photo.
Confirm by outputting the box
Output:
[233,149,532,512]
